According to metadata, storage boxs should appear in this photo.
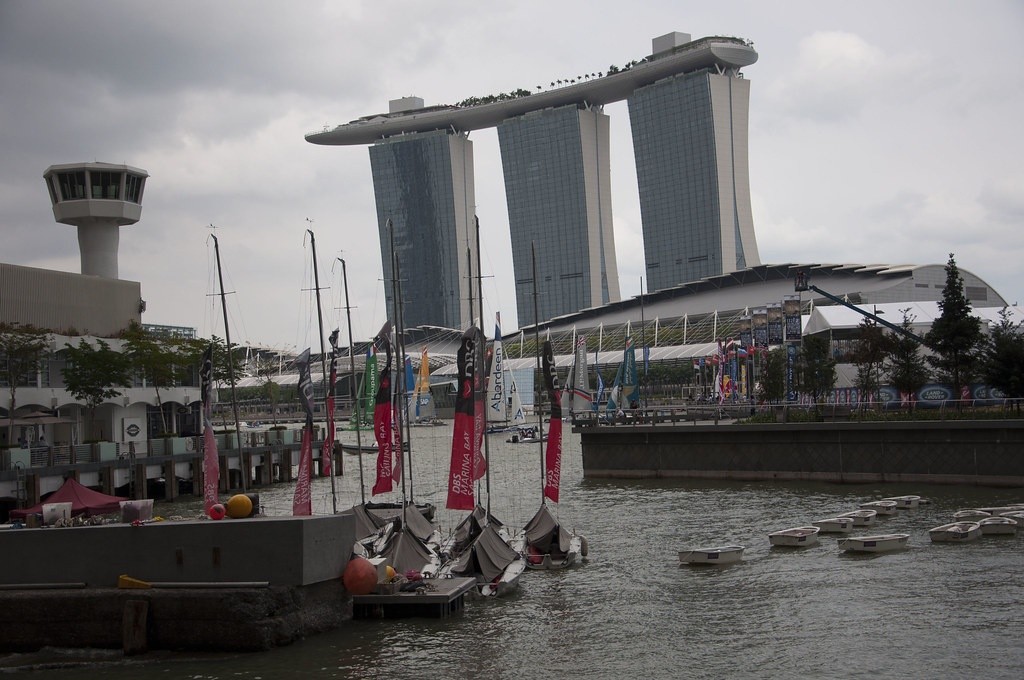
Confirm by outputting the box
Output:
[118,499,154,523]
[42,501,72,524]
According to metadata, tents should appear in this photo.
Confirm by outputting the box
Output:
[9,478,128,524]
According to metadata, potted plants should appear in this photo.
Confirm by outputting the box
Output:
[215,429,245,450]
[264,426,294,446]
[301,424,321,441]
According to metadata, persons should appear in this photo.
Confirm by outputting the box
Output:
[18,436,49,448]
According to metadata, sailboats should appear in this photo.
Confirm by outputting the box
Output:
[318,213,594,597]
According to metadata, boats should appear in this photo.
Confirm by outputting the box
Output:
[953,510,992,521]
[882,495,921,509]
[837,509,877,526]
[980,517,1018,535]
[979,503,1024,528]
[678,545,746,565]
[859,501,897,515]
[837,532,911,553]
[928,520,981,543]
[768,525,821,547]
[811,517,855,533]
